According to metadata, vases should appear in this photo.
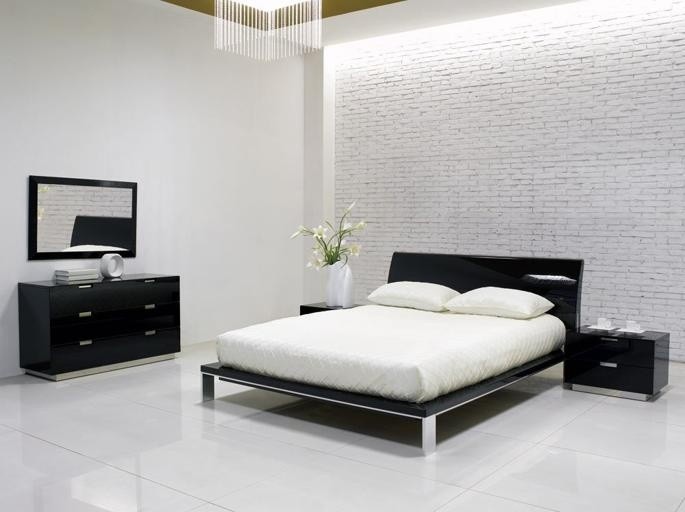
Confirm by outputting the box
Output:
[327,262,356,309]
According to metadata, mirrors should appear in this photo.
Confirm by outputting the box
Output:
[28,175,137,261]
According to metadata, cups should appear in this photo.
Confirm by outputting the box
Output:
[627,321,639,330]
[598,318,611,328]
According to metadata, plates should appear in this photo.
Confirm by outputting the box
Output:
[589,324,618,331]
[620,326,645,336]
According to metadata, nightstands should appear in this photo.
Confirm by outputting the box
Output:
[563,325,671,402]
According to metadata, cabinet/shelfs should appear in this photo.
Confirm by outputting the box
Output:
[18,273,182,382]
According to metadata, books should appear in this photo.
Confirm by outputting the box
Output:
[55,269,102,285]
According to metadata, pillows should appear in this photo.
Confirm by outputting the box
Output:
[365,279,556,318]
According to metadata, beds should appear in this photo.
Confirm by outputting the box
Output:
[198,250,585,458]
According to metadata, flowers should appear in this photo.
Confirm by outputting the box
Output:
[290,200,371,274]
